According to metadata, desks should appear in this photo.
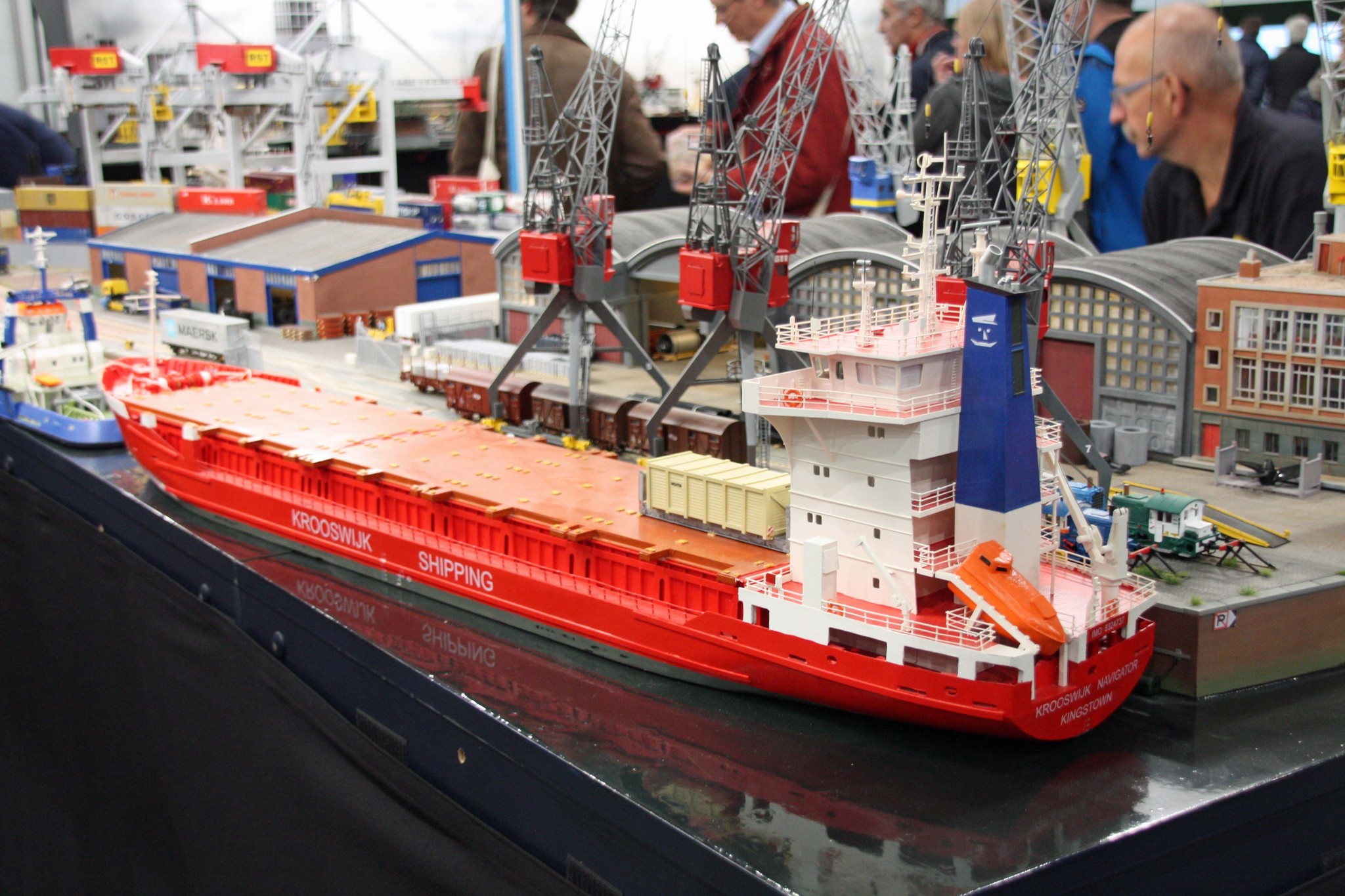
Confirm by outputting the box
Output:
[0,421,1345,896]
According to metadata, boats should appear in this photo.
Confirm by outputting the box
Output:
[1,224,130,450]
[100,212,1161,746]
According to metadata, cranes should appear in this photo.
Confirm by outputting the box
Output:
[641,0,853,469]
[929,1,1113,555]
[488,0,676,451]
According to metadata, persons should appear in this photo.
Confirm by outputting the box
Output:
[676,1,863,218]
[873,0,1345,263]
[449,1,665,212]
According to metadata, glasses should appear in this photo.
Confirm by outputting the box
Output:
[1113,73,1192,107]
[713,0,737,17]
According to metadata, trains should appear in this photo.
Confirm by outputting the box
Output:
[1040,470,1224,576]
[395,337,749,466]
